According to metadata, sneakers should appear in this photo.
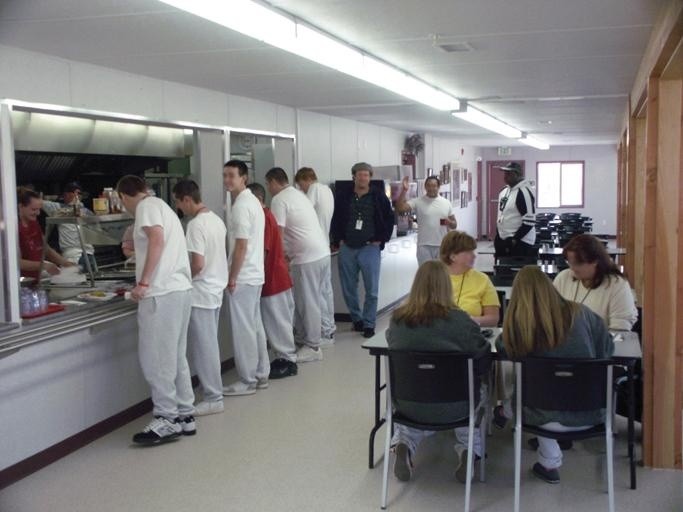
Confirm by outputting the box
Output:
[222,378,256,395]
[493,405,506,429]
[364,328,374,337]
[352,320,362,331]
[529,437,573,450]
[454,448,475,484]
[194,400,224,416]
[256,376,267,388]
[533,462,561,484]
[394,441,412,481]
[320,336,335,345]
[133,415,182,443]
[296,346,323,363]
[269,363,289,378]
[178,415,195,435]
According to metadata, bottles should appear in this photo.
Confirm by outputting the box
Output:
[102,187,116,214]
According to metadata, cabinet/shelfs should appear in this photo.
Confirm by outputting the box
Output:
[331,230,419,322]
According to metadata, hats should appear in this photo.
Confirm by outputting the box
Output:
[499,162,521,174]
[352,162,373,175]
[63,182,80,192]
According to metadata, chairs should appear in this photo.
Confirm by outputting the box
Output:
[514,357,615,512]
[381,349,486,511]
[487,291,505,438]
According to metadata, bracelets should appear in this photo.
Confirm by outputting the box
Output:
[137,282,149,288]
[229,284,235,287]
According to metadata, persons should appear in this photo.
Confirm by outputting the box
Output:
[117,175,198,444]
[295,167,337,347]
[329,163,395,338]
[16,186,77,289]
[389,260,494,484]
[221,159,271,397]
[394,176,458,268]
[244,183,299,379]
[438,230,501,327]
[554,234,638,331]
[494,162,540,264]
[122,183,156,257]
[171,179,229,417]
[41,181,95,273]
[492,266,615,484]
[264,169,330,364]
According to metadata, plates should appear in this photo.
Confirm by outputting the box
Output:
[88,271,135,279]
[50,266,86,283]
[23,304,64,320]
[78,292,117,301]
